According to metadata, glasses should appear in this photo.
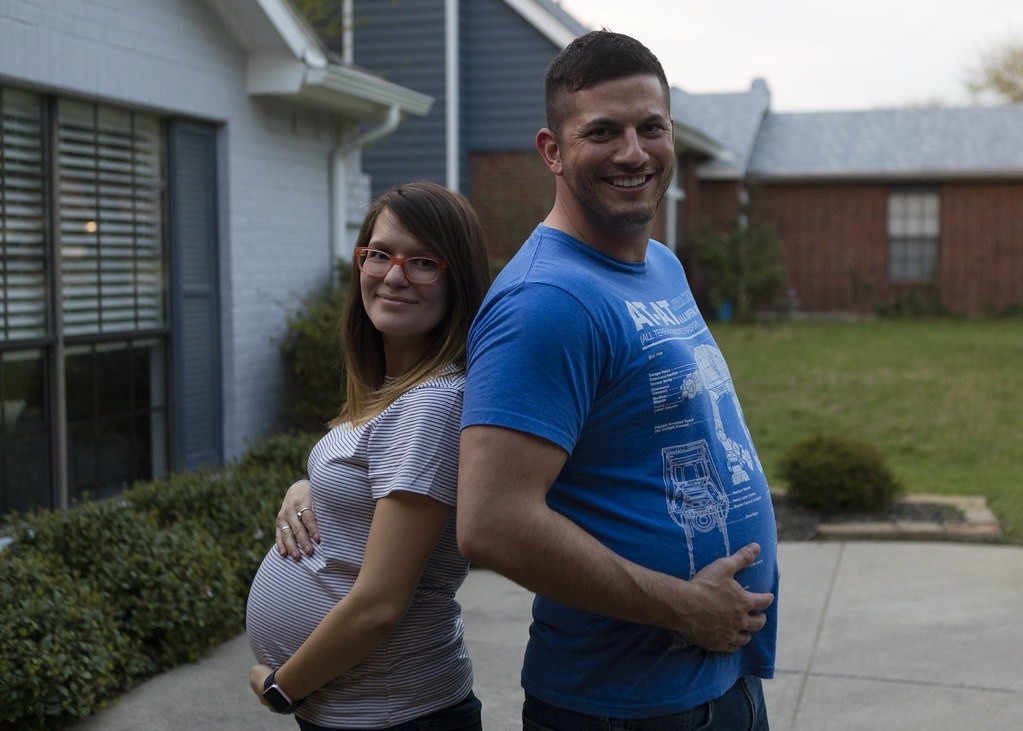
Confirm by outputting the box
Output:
[354,247,449,285]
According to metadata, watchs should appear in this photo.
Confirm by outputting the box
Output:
[262,669,307,714]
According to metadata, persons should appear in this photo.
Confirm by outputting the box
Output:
[455,40,783,731]
[245,180,494,731]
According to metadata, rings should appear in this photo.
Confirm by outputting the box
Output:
[298,507,314,520]
[281,525,290,532]
